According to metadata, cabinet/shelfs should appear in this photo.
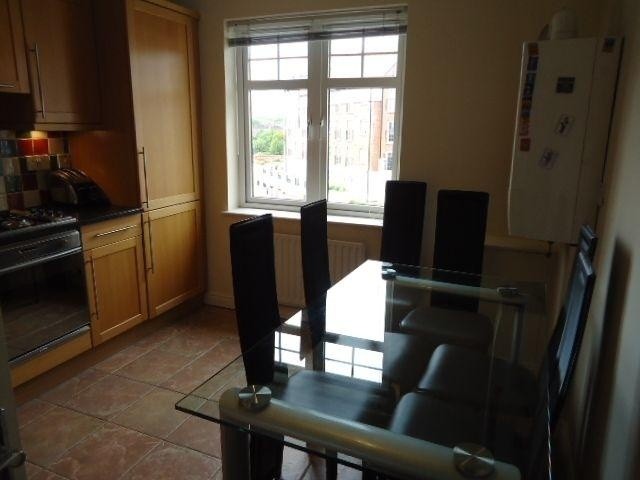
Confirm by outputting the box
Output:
[67,0,204,212]
[80,212,149,348]
[20,0,113,131]
[0,0,31,94]
[141,200,208,324]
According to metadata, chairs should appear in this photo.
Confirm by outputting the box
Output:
[300,198,418,384]
[390,237,632,477]
[230,212,395,479]
[378,179,427,328]
[418,223,599,389]
[398,189,492,353]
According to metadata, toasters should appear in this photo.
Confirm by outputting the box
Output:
[42,168,96,210]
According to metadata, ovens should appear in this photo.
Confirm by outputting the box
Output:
[1,218,90,367]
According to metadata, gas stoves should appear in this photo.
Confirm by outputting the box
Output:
[1,206,79,241]
